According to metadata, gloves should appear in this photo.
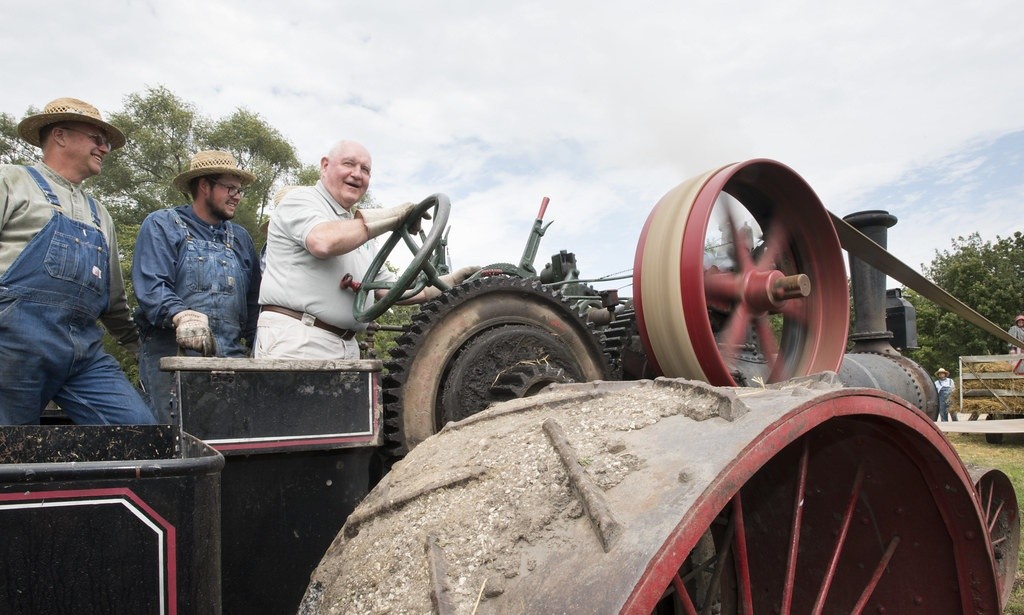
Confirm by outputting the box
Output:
[424,265,482,302]
[173,310,218,357]
[117,337,145,355]
[354,201,432,239]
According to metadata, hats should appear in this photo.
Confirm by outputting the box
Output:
[172,150,256,193]
[17,97,126,151]
[934,368,950,378]
[1015,314,1024,322]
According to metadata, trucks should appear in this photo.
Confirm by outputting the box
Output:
[958,354,1024,443]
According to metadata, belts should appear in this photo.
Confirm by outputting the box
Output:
[260,305,356,341]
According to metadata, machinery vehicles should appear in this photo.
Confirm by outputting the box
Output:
[0,157,1023,615]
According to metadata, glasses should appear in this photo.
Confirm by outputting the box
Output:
[206,176,245,197]
[60,126,111,153]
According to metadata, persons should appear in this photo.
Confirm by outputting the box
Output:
[130,150,262,425]
[0,97,157,427]
[934,368,957,422]
[252,140,480,359]
[1008,315,1024,354]
